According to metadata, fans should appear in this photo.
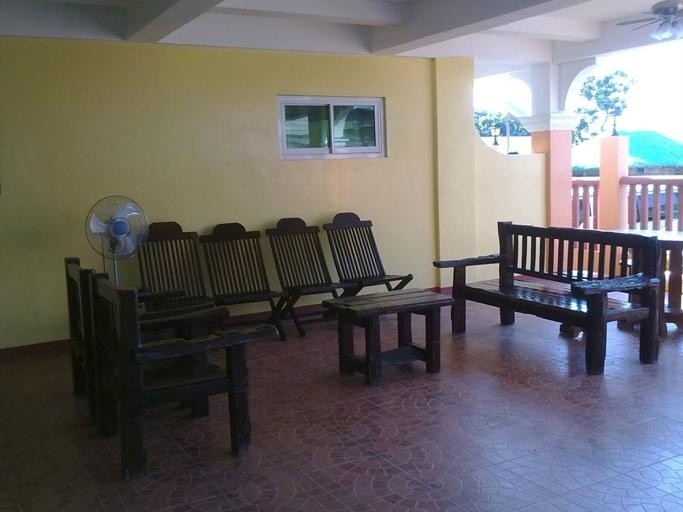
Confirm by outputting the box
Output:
[85,195,149,291]
[616,0,683,32]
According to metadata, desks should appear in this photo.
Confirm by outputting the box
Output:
[617,230,683,331]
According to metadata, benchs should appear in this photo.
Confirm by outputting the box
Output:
[434,222,664,376]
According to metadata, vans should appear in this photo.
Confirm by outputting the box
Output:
[571,185,678,231]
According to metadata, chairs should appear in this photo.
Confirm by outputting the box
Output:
[199,223,287,341]
[265,217,359,338]
[64,257,217,410]
[138,221,214,313]
[88,273,280,481]
[323,212,414,299]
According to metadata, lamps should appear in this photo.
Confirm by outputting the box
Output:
[650,15,683,40]
